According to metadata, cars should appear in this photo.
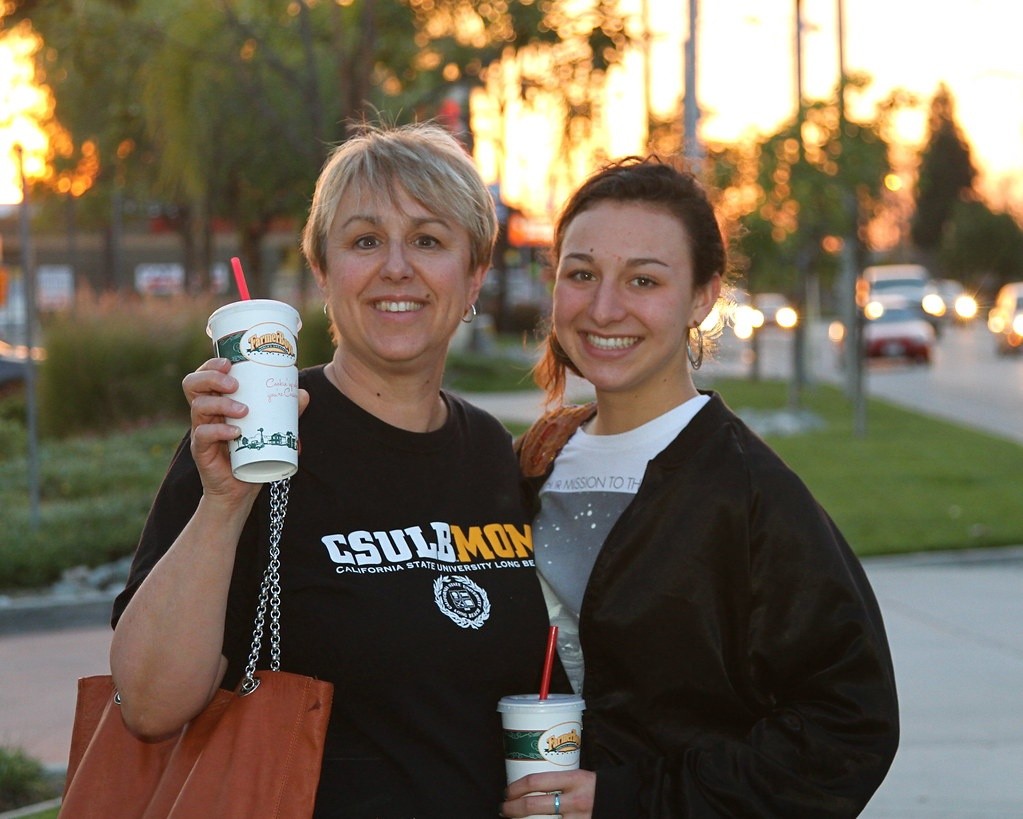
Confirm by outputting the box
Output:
[748,296,794,330]
[990,284,1023,359]
[932,281,975,325]
[856,297,938,364]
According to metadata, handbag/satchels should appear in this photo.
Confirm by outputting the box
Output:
[54,478,333,819]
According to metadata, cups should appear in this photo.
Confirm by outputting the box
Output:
[205,299,304,482]
[496,694,586,819]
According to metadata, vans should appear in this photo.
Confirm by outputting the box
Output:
[856,265,939,317]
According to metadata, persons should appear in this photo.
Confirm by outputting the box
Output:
[112,126,569,819]
[503,154,900,819]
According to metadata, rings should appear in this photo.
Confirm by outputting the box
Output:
[554,793,559,814]
